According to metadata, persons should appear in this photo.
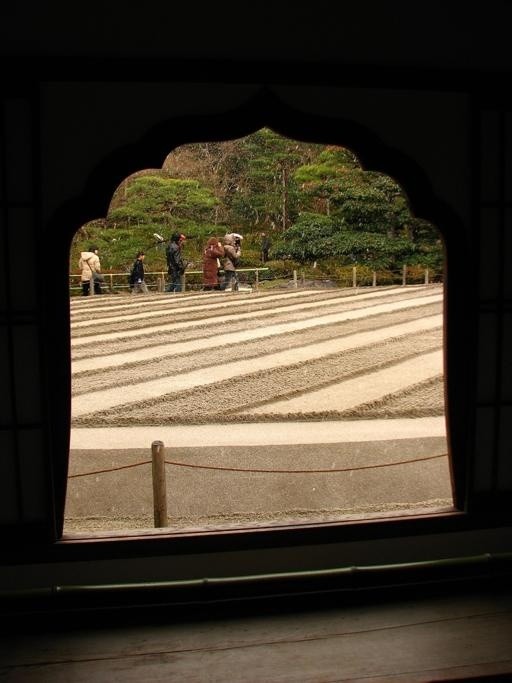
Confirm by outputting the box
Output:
[165,232,185,292]
[218,232,243,290]
[131,251,149,294]
[200,236,225,293]
[260,232,270,261]
[78,245,102,295]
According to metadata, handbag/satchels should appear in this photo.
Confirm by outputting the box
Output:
[92,271,104,281]
